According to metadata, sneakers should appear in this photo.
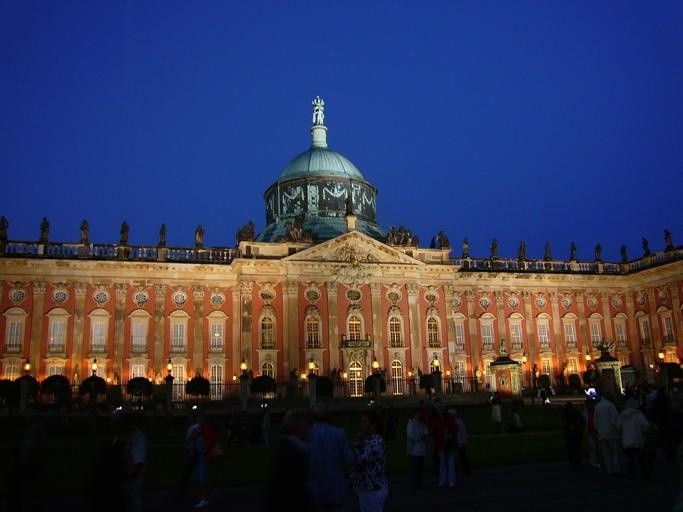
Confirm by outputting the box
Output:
[192,500,209,509]
[568,461,657,489]
[448,482,457,487]
[436,479,446,488]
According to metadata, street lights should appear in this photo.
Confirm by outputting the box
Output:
[89,358,97,375]
[305,356,316,408]
[24,358,30,376]
[585,350,591,367]
[371,355,382,406]
[657,352,665,368]
[237,357,248,412]
[164,357,174,403]
[433,355,442,400]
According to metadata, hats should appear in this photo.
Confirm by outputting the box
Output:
[447,408,457,416]
[413,411,426,421]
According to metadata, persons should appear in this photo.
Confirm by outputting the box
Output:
[118,221,128,246]
[597,337,615,353]
[383,224,449,248]
[543,240,553,260]
[40,216,49,243]
[462,237,470,258]
[499,344,508,356]
[490,238,499,259]
[0,216,8,250]
[619,244,627,263]
[663,228,674,252]
[158,223,168,245]
[594,241,601,262]
[517,239,526,261]
[237,219,312,248]
[80,218,88,246]
[194,224,204,246]
[569,241,577,263]
[640,237,651,258]
